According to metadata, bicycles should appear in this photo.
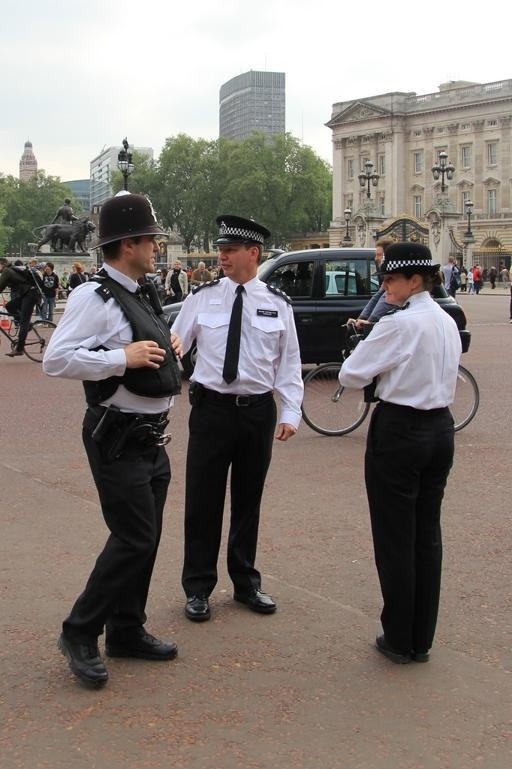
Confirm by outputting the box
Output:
[301,316,478,436]
[1,307,59,363]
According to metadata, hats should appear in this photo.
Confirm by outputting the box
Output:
[89,192,171,250]
[372,241,441,276]
[211,214,272,244]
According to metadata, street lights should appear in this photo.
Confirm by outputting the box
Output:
[356,160,379,198]
[119,139,135,193]
[343,206,352,242]
[431,151,456,192]
[463,200,474,243]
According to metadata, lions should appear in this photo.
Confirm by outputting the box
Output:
[32,216,96,253]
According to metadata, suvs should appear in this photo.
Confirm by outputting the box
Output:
[163,248,471,380]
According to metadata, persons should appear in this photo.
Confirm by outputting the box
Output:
[0,256,37,356]
[438,255,511,298]
[337,244,464,666]
[166,215,306,622]
[10,258,224,329]
[41,196,186,689]
[49,198,80,225]
[346,237,400,343]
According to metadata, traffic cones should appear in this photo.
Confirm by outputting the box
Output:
[1,299,12,330]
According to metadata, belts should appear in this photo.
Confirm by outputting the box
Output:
[191,387,274,406]
[86,407,169,424]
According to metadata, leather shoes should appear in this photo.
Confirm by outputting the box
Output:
[110,627,178,661]
[375,632,431,664]
[55,633,108,690]
[5,349,24,356]
[233,586,277,614]
[184,588,212,622]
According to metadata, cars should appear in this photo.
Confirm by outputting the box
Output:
[326,270,381,295]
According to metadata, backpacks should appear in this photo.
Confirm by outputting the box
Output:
[450,266,462,291]
[9,264,43,290]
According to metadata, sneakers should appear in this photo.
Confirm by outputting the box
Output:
[43,324,49,328]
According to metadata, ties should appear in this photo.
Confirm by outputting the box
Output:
[221,286,250,386]
